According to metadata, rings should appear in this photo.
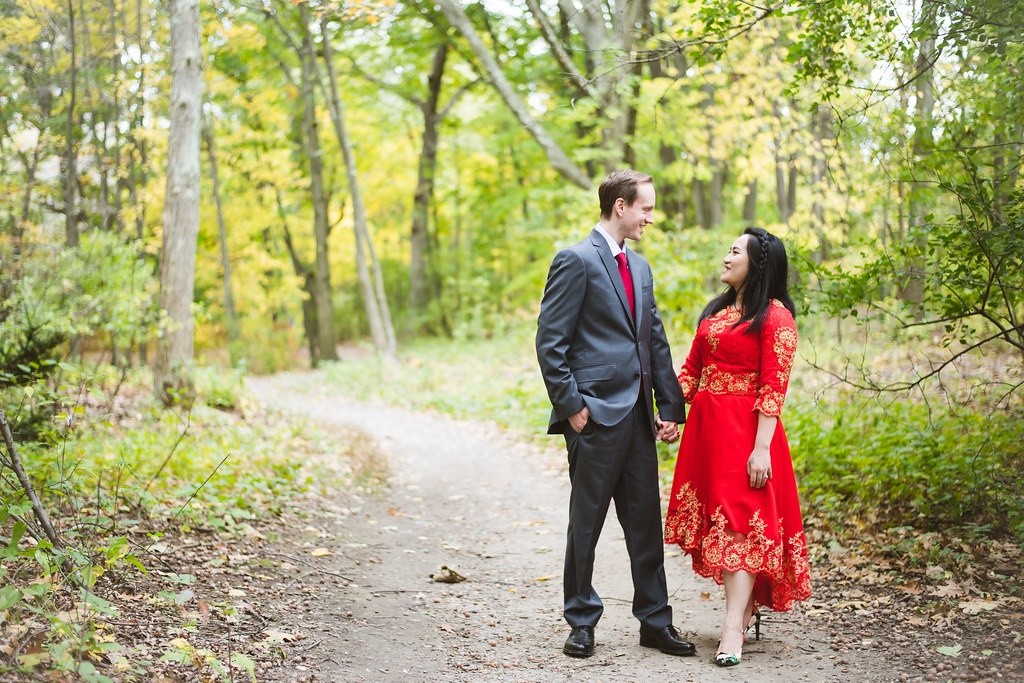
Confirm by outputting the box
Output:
[763,474,768,479]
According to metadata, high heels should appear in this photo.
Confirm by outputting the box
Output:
[716,606,761,647]
[715,649,742,665]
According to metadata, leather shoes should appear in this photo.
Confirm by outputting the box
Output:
[563,625,594,655]
[639,625,695,655]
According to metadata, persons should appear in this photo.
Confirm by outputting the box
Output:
[534,168,695,660]
[658,227,815,667]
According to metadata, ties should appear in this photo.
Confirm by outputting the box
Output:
[614,252,635,324]
